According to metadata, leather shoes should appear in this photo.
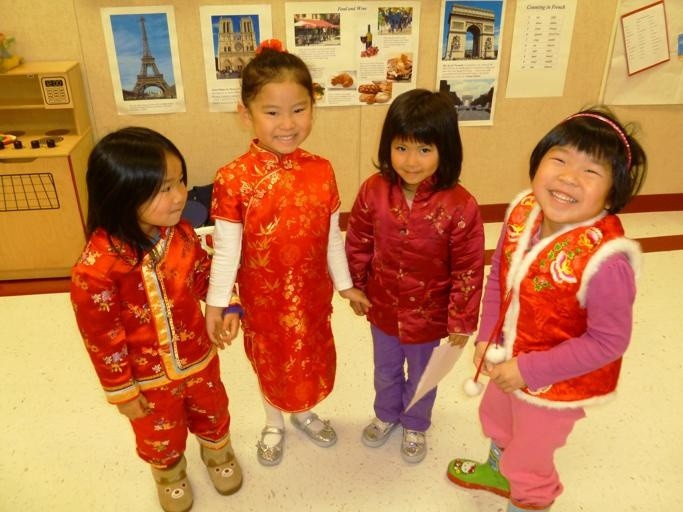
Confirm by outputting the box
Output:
[362,419,393,447]
[257,427,285,466]
[291,413,338,446]
[402,428,426,462]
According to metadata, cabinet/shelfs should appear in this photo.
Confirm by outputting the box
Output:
[0,59,96,282]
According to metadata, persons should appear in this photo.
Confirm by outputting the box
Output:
[202,36,375,468]
[448,101,649,511]
[68,127,245,512]
[344,87,488,467]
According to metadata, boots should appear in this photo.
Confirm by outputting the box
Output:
[153,456,193,512]
[446,442,511,497]
[200,442,243,495]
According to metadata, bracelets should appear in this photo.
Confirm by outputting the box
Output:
[220,305,243,320]
